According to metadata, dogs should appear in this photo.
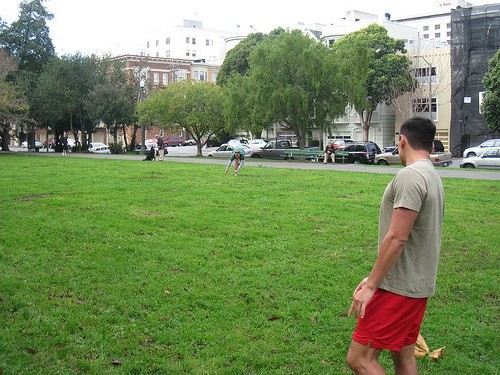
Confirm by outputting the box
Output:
[142,146,157,161]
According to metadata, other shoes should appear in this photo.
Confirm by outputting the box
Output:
[325,163,327,164]
[333,162,334,165]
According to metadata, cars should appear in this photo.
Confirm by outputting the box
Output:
[221,139,249,146]
[21,139,43,148]
[88,142,110,154]
[139,139,158,149]
[459,149,500,169]
[336,141,381,164]
[184,137,199,146]
[249,139,271,147]
[62,139,78,150]
[207,144,252,158]
[463,139,500,157]
[42,138,57,149]
[163,137,184,147]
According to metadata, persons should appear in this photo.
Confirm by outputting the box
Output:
[154,134,165,161]
[346,116,445,375]
[225,146,245,176]
[323,142,335,165]
[61,131,68,156]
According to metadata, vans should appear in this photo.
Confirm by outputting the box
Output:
[385,140,444,152]
[277,135,309,148]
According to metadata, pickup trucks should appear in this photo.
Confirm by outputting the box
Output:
[373,147,452,165]
[249,140,320,160]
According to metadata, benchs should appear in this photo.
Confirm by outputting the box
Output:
[280,150,349,163]
